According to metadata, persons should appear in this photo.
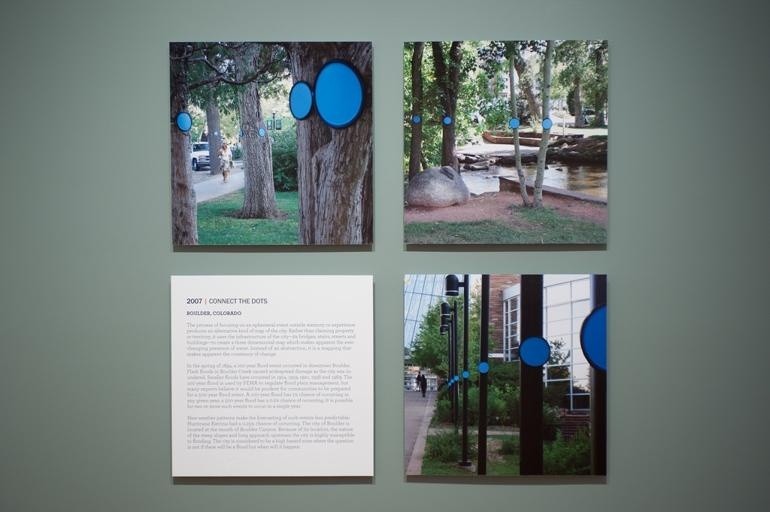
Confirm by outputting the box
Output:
[217,142,233,181]
[415,371,422,392]
[418,374,427,397]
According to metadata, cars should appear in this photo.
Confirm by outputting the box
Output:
[581,109,597,125]
[470,113,484,127]
[558,115,591,128]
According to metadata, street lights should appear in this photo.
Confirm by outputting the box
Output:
[433,273,475,467]
[265,109,284,137]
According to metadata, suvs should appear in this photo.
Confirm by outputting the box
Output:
[190,139,212,171]
